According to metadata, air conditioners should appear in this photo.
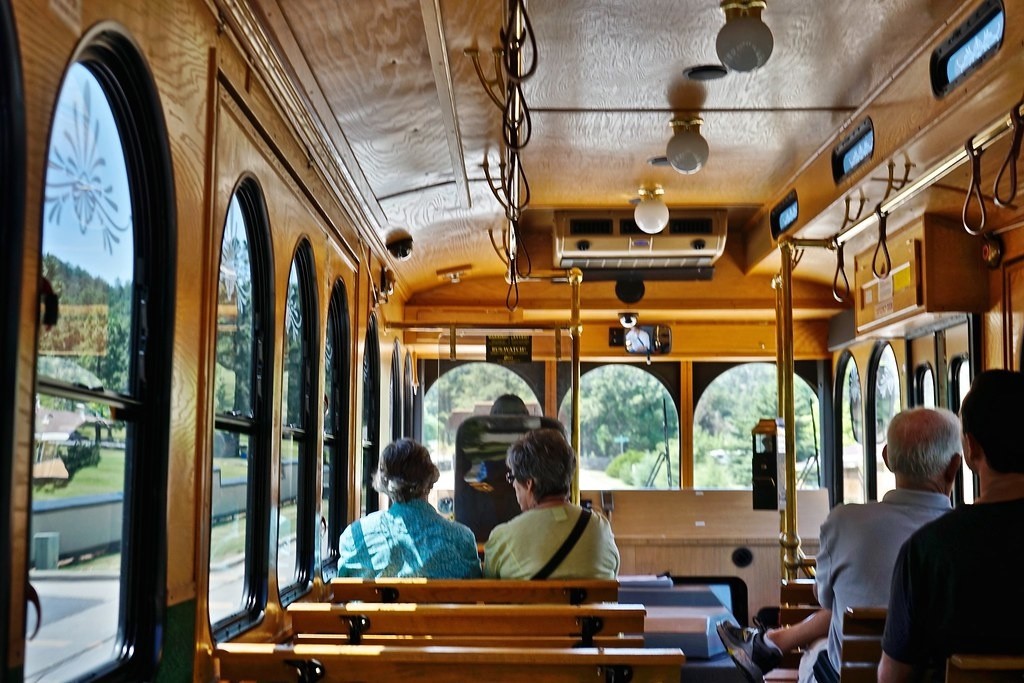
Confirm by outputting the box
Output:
[550,210,728,269]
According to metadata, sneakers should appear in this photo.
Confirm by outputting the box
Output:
[715,616,784,683]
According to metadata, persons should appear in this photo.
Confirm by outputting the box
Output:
[488,393,530,416]
[716,406,964,683]
[483,428,621,581]
[336,438,483,579]
[877,368,1024,683]
[762,438,773,453]
[626,325,650,352]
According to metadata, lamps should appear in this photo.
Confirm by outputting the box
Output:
[716,0,774,73]
[666,118,710,174]
[633,188,670,234]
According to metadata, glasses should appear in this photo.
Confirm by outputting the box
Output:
[506,472,518,484]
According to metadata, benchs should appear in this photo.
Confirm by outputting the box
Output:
[212,578,1024,683]
